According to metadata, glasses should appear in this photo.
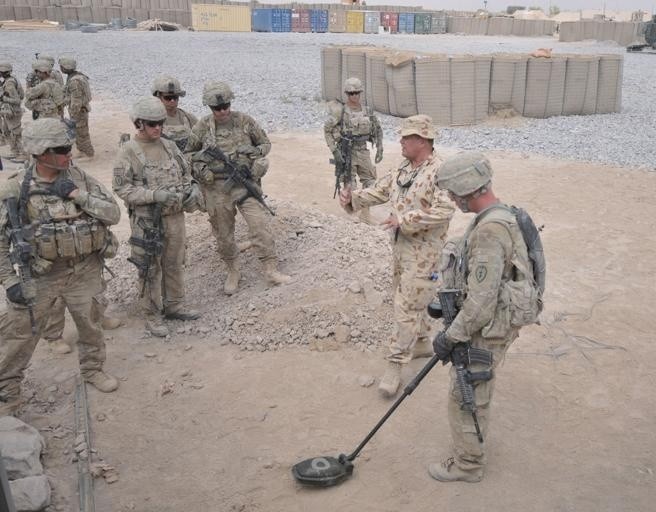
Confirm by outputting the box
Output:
[348,91,361,96]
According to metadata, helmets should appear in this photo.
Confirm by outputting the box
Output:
[344,77,363,94]
[0,52,234,157]
[433,151,494,198]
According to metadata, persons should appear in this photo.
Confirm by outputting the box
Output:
[183,81,293,296]
[338,113,456,397]
[0,119,118,417]
[41,297,121,355]
[425,149,546,483]
[323,77,384,224]
[0,52,94,163]
[111,95,202,338]
[150,73,206,266]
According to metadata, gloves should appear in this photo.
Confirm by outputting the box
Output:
[192,149,214,164]
[333,149,346,164]
[236,143,260,157]
[432,330,457,360]
[374,150,383,164]
[6,283,26,305]
[46,177,79,199]
[182,182,200,208]
[153,188,179,207]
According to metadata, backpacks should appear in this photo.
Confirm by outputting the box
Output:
[440,230,471,298]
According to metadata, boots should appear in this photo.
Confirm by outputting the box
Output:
[377,360,402,398]
[360,206,377,227]
[222,256,243,295]
[412,334,434,360]
[261,257,291,286]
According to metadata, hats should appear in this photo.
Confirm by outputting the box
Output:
[393,114,441,140]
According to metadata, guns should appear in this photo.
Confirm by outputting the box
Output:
[206,146,276,217]
[329,137,349,199]
[436,288,492,444]
[3,197,37,338]
[128,203,165,299]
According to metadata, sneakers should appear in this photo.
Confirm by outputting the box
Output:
[82,370,119,394]
[142,306,203,337]
[74,149,94,162]
[47,335,72,355]
[428,457,485,484]
[100,314,121,330]
[3,152,29,164]
